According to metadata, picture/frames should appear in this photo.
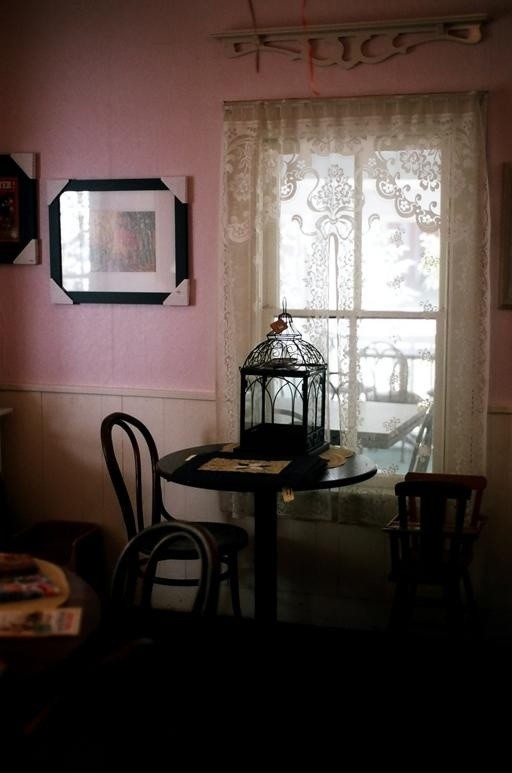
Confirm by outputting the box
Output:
[46,173,194,312]
[2,145,46,270]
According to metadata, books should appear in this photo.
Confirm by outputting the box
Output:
[1,605,85,635]
[1,564,61,604]
